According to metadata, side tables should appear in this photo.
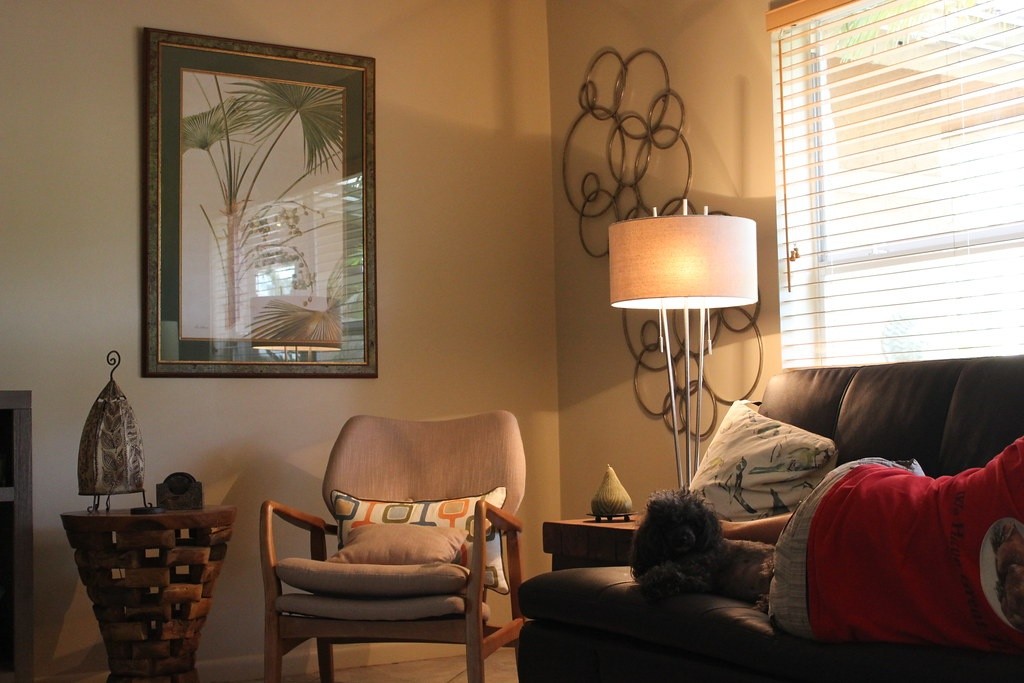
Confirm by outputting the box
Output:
[542,513,644,572]
[59,504,237,682]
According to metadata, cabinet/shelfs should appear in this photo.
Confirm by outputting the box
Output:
[0,389,37,683]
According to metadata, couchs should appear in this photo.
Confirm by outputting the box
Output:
[517,353,1024,683]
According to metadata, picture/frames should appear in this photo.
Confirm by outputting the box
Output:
[142,25,380,379]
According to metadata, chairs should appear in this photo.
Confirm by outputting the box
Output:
[257,412,528,683]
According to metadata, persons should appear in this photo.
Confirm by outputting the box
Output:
[720,434,1024,656]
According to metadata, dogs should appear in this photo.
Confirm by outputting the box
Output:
[628,481,775,617]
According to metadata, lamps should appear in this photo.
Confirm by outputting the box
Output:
[608,198,759,490]
